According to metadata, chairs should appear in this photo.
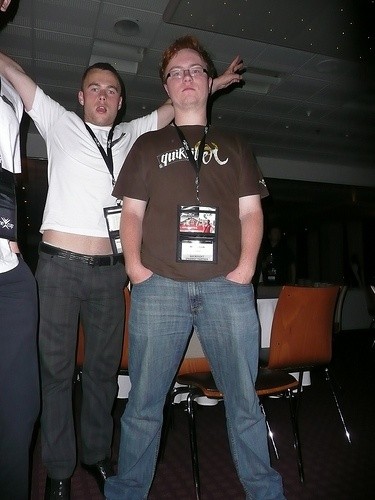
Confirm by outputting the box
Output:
[77,282,375,500]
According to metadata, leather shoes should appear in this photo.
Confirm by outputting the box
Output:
[44,476,71,500]
[80,458,113,499]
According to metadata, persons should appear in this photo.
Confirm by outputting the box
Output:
[0,0,41,500]
[104,36,284,500]
[1,53,244,500]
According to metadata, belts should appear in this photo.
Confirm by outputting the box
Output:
[38,240,121,267]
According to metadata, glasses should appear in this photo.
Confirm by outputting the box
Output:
[165,67,212,81]
